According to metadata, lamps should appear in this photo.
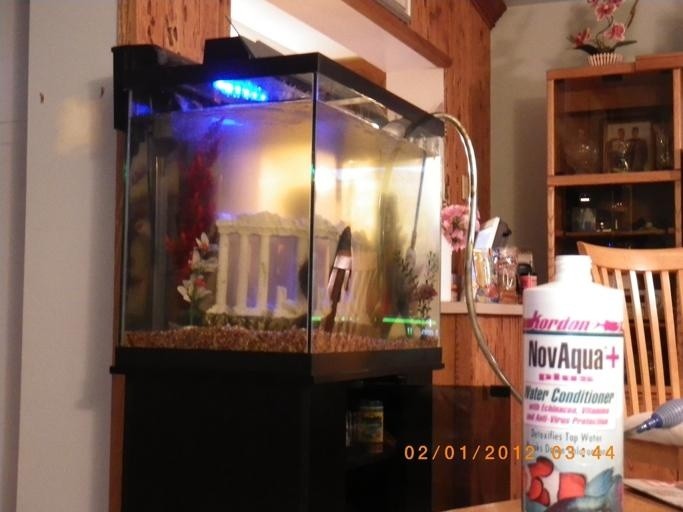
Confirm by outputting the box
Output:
[573,237,682,490]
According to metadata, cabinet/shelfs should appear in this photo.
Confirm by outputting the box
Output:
[563,128,598,172]
[624,398,683,439]
[359,404,384,455]
[519,252,627,512]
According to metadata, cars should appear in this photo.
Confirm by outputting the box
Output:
[472,247,536,306]
[604,139,631,171]
[571,206,596,235]
[653,123,673,170]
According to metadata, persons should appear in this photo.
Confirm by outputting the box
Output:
[606,129,631,170]
[625,127,648,172]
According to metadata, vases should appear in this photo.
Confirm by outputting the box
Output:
[439,202,484,257]
[561,0,644,56]
[175,231,222,325]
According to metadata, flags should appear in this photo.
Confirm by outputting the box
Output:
[584,51,625,69]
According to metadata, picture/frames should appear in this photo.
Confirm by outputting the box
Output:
[440,478,683,511]
[101,343,454,512]
[421,296,524,512]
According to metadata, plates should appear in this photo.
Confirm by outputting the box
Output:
[537,51,682,426]
